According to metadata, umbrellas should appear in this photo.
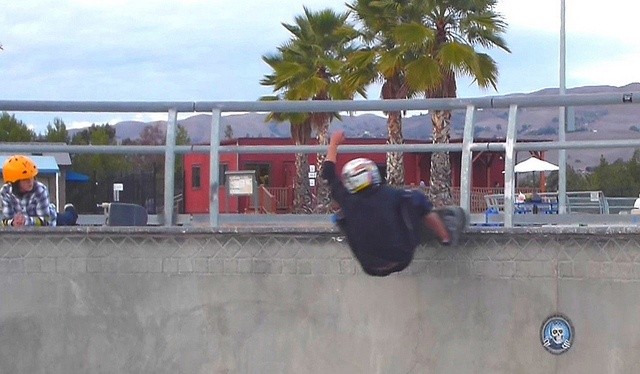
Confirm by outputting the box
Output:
[502,157,560,186]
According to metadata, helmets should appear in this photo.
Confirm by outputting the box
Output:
[2,155,38,185]
[341,157,382,194]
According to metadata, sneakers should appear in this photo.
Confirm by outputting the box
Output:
[439,208,460,249]
[64,203,76,226]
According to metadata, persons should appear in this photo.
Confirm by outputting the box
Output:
[634,193,640,207]
[1,155,78,228]
[322,132,464,277]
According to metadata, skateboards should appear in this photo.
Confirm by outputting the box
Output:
[334,207,467,244]
